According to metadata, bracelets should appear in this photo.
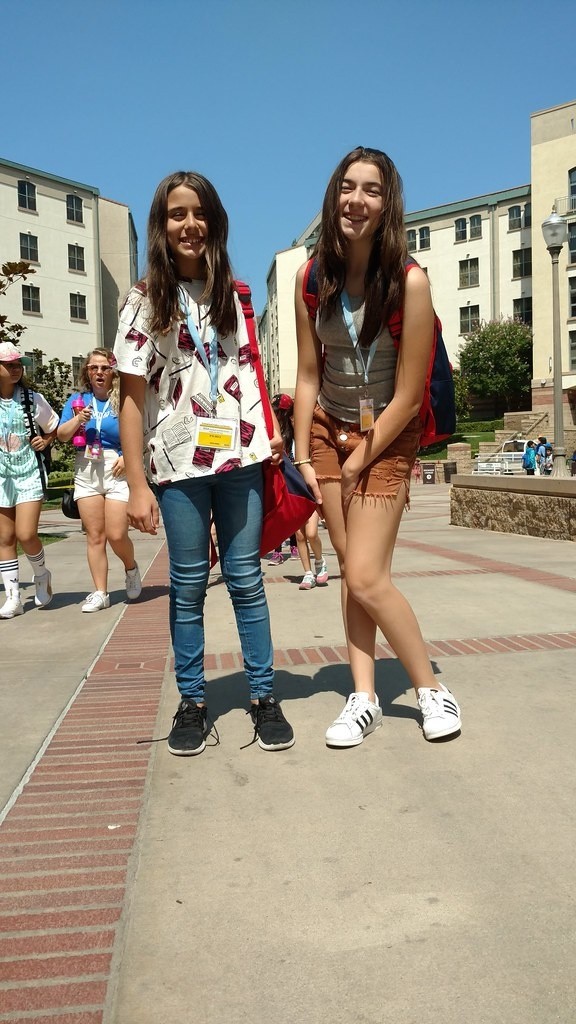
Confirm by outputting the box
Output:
[292,460,311,465]
[77,415,80,422]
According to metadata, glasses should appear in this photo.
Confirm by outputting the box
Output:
[86,364,114,373]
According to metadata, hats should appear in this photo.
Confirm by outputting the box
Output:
[269,393,293,411]
[0,342,27,362]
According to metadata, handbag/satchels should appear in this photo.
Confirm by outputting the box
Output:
[62,489,81,519]
[259,449,317,559]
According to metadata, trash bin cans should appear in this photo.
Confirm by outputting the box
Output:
[423,464,435,484]
[443,462,457,483]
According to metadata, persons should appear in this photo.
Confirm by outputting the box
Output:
[57,348,142,612]
[206,392,329,591]
[0,341,59,618]
[413,458,421,483]
[525,434,554,476]
[109,172,293,756]
[269,146,461,747]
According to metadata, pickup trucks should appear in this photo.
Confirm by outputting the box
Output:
[476,440,538,475]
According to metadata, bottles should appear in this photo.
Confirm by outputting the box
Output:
[71,396,86,446]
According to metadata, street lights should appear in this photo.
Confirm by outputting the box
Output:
[541,205,572,478]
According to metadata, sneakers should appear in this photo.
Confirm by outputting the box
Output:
[125,560,142,599]
[240,693,295,751]
[314,557,328,585]
[0,589,24,619]
[290,546,302,561]
[31,569,53,607]
[325,692,383,746]
[81,591,111,613]
[417,682,462,741]
[268,551,285,566]
[137,699,221,756]
[298,571,316,590]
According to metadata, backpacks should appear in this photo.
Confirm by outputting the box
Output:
[24,387,51,493]
[522,451,533,468]
[302,254,457,448]
[540,445,548,458]
